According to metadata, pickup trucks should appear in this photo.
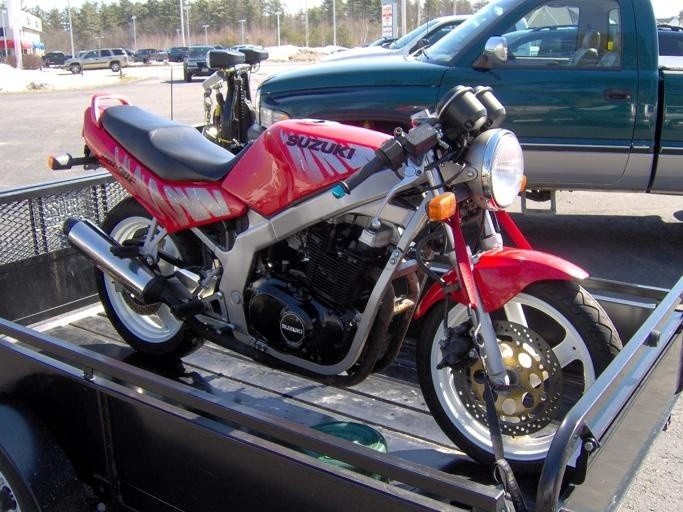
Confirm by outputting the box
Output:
[255,0,683,196]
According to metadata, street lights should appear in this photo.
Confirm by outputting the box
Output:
[238,19,246,44]
[202,24,209,44]
[132,15,137,49]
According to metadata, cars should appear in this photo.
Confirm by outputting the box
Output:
[40,44,269,82]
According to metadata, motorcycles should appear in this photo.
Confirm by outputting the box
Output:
[48,84,625,477]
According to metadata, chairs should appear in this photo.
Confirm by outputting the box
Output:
[597,33,622,68]
[565,30,601,66]
[550,36,562,51]
